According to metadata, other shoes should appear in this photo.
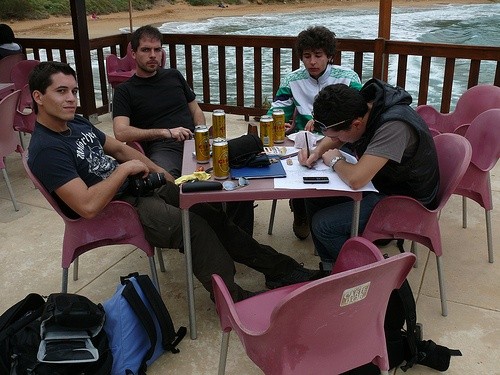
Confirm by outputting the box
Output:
[266,262,331,289]
[288,198,310,241]
[236,284,268,301]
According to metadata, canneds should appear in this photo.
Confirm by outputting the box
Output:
[194,125,210,164]
[212,110,226,141]
[272,109,285,144]
[260,116,274,147]
[212,139,229,180]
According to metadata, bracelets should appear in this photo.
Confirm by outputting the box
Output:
[331,156,346,172]
[168,128,172,138]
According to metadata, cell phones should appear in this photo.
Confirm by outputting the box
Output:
[303,177,329,183]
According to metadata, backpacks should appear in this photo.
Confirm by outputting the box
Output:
[102,272,187,375]
[0,293,113,375]
[338,254,462,375]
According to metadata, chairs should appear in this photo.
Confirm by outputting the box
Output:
[22,153,166,299]
[106,42,167,153]
[212,85,500,375]
[0,53,42,212]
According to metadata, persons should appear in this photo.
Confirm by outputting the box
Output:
[0,23,22,59]
[92,12,99,19]
[298,79,440,279]
[29,61,334,306]
[263,26,363,240]
[112,27,255,240]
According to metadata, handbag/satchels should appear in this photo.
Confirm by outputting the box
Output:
[206,134,264,173]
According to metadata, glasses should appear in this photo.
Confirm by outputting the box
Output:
[311,110,351,132]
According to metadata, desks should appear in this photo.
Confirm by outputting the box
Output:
[179,140,362,341]
